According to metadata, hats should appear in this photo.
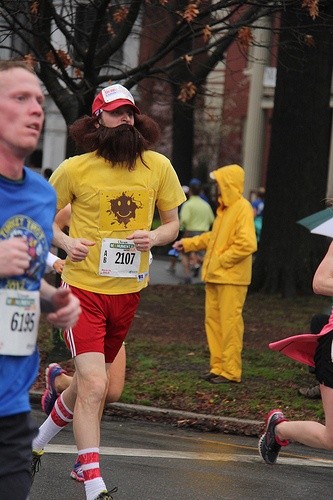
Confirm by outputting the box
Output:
[92,84,141,116]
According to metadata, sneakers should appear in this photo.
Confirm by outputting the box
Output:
[70,460,84,481]
[42,362,67,415]
[258,409,290,465]
[32,448,45,480]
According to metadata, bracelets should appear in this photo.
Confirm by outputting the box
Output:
[46,251,60,267]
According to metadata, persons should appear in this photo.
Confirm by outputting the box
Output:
[0,64,82,500]
[166,186,194,274]
[42,202,152,417]
[178,183,215,276]
[172,165,257,383]
[250,188,266,241]
[258,241,333,463]
[297,313,332,398]
[31,84,186,499]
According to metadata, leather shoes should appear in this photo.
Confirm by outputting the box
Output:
[198,371,241,384]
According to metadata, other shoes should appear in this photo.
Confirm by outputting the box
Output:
[298,384,322,399]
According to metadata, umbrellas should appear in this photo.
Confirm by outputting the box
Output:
[295,205,333,239]
